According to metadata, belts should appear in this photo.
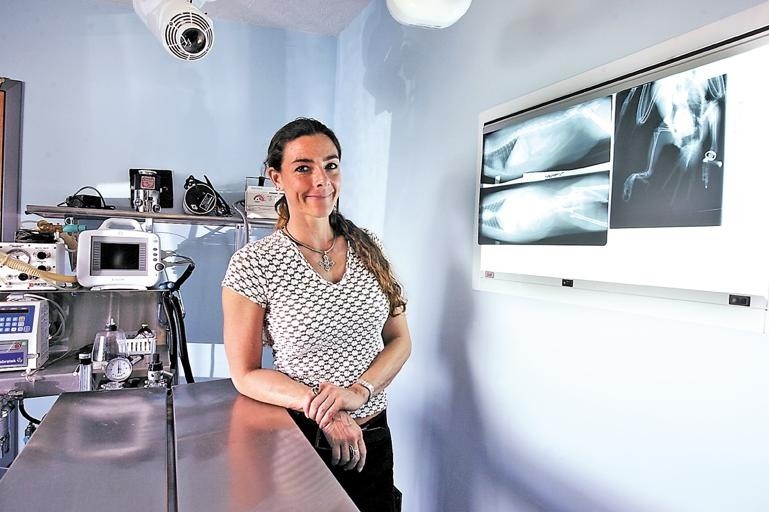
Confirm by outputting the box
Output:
[310,424,389,452]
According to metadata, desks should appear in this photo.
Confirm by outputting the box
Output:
[0,378,361,512]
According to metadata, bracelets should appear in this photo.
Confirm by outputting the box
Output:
[356,378,374,400]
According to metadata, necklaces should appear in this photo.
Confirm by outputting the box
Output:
[284,222,336,272]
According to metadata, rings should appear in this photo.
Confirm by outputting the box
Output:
[349,444,360,456]
[310,383,320,395]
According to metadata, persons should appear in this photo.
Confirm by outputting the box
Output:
[224,116,413,512]
[225,394,297,512]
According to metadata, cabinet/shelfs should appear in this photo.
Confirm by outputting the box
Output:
[1,204,278,399]
